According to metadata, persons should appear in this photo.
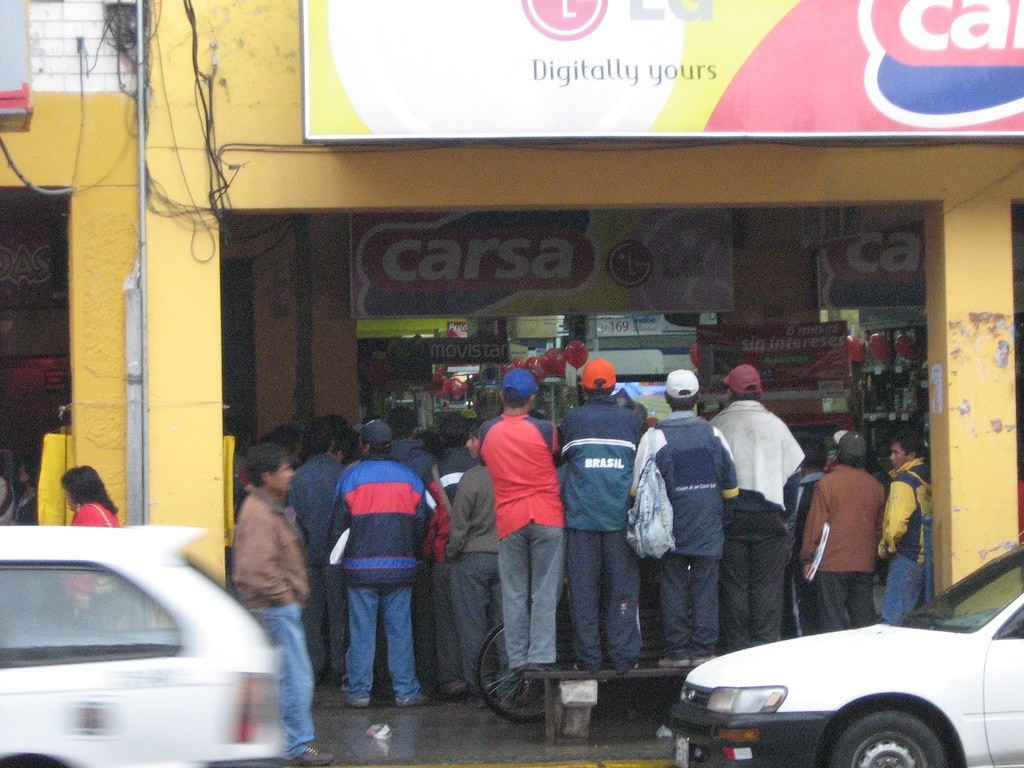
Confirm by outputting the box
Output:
[290,416,347,684]
[800,431,884,634]
[477,369,564,674]
[387,406,436,488]
[328,421,436,711]
[62,465,120,527]
[793,435,828,639]
[447,422,508,708]
[631,369,739,667]
[706,364,806,652]
[234,443,335,764]
[419,418,483,697]
[553,359,646,673]
[17,457,41,525]
[878,431,931,626]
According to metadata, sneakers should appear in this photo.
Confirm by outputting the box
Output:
[287,748,334,766]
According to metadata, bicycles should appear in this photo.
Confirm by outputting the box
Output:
[473,576,575,724]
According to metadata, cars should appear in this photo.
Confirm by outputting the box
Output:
[669,542,1024,768]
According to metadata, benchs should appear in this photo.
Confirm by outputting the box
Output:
[522,661,695,740]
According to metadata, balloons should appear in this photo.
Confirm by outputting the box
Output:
[439,339,587,400]
[843,334,915,362]
[690,343,698,367]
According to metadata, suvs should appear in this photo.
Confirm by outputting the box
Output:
[0,524,291,768]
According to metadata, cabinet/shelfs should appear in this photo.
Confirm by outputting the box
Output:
[846,316,935,470]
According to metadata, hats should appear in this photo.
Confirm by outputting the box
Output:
[666,369,699,399]
[501,369,539,399]
[581,358,617,390]
[354,419,394,446]
[723,365,763,394]
[834,431,866,455]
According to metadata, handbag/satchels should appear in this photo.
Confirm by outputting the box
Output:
[629,428,676,560]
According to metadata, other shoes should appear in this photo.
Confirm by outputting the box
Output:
[514,664,528,673]
[528,662,561,671]
[658,657,712,667]
[346,698,368,709]
[396,694,433,707]
[468,696,487,708]
